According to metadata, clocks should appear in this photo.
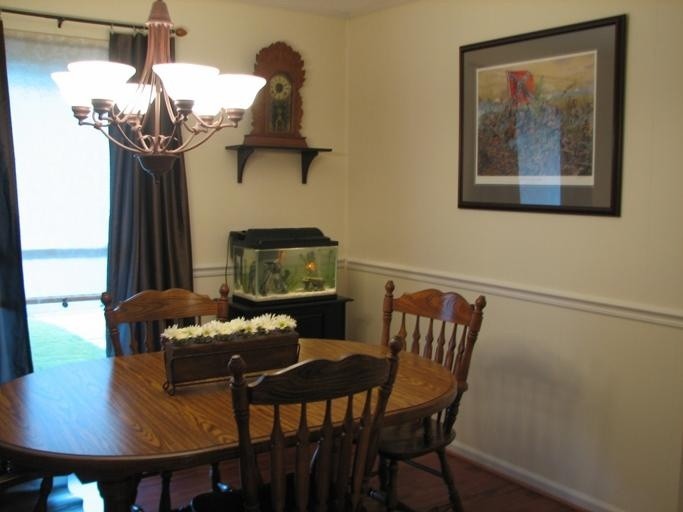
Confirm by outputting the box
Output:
[243,41,307,147]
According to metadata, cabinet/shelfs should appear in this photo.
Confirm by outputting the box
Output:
[230,295,354,340]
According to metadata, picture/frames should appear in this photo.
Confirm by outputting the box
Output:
[457,13,627,217]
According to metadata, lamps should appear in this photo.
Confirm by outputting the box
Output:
[50,0,267,185]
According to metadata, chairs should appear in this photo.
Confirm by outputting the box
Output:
[181,337,403,512]
[365,280,487,512]
[101,282,229,357]
[0,460,84,512]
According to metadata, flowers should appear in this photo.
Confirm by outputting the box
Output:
[159,312,298,340]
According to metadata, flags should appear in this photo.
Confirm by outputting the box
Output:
[505,70,536,104]
